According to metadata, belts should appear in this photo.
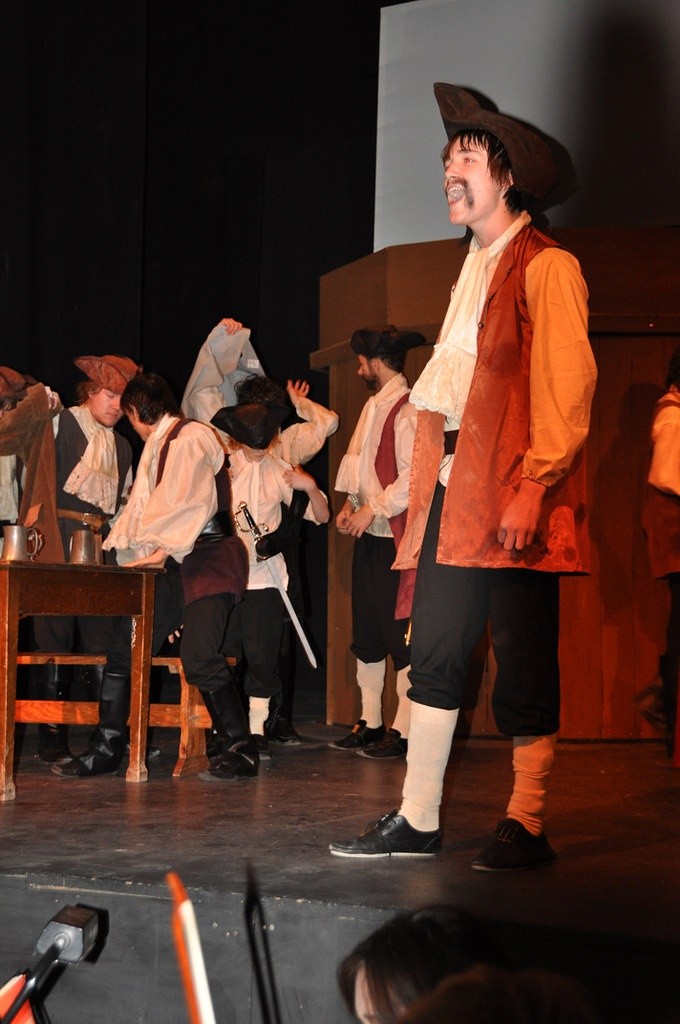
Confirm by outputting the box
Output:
[56,508,110,532]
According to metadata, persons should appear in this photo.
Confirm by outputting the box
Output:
[639,344,680,760]
[327,324,431,762]
[340,905,600,1024]
[0,317,330,782]
[327,82,597,874]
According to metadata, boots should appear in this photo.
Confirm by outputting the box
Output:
[50,667,131,777]
[264,681,304,745]
[195,671,260,780]
[35,663,75,767]
[156,686,181,772]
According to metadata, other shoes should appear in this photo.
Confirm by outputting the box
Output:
[636,682,669,735]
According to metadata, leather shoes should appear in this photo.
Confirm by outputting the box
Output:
[327,808,443,858]
[355,728,407,760]
[470,817,554,872]
[327,719,387,750]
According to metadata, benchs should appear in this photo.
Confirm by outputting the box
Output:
[15,653,237,777]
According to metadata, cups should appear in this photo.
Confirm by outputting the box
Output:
[67,529,104,565]
[0,524,44,562]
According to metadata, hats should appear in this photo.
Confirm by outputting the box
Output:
[210,402,294,451]
[351,324,429,359]
[433,82,561,199]
[73,354,142,395]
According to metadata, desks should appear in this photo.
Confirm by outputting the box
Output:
[0,560,167,801]
